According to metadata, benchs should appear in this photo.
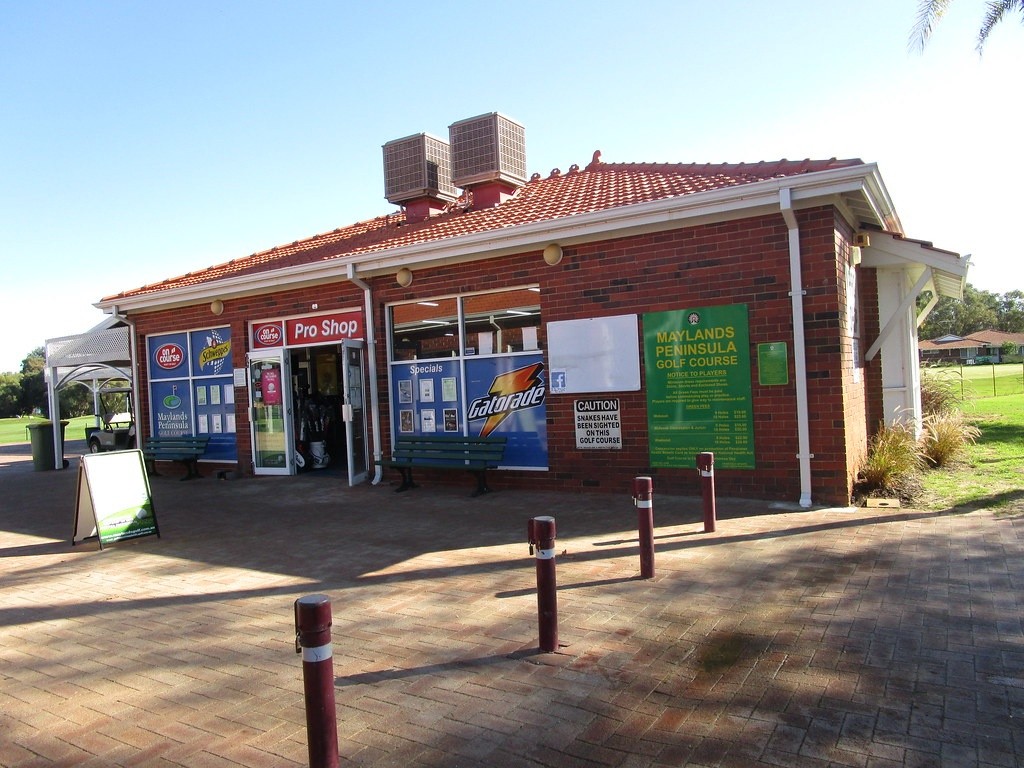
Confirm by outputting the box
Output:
[373,436,507,496]
[143,436,210,481]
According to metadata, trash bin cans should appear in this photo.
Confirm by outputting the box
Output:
[26,421,70,472]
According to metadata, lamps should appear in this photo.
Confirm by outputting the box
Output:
[211,301,223,316]
[542,244,563,266]
[397,268,414,287]
